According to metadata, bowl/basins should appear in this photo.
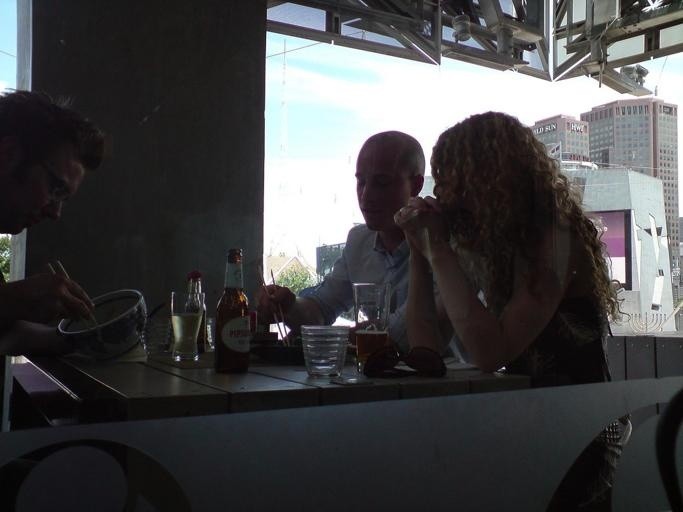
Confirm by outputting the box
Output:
[58,288,148,361]
[265,342,302,365]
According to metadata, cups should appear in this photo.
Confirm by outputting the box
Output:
[204,315,217,352]
[351,281,392,380]
[169,290,206,365]
[300,324,351,377]
[136,313,178,360]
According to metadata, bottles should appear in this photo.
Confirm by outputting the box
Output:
[215,247,251,375]
[183,269,207,355]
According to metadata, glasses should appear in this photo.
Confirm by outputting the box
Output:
[34,154,72,207]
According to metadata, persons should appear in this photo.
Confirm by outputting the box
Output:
[249,130,480,363]
[0,90,110,364]
[394,113,625,511]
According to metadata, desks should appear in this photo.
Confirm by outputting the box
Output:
[54,351,533,418]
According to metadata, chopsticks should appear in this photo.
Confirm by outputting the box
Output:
[256,265,290,348]
[47,258,98,329]
